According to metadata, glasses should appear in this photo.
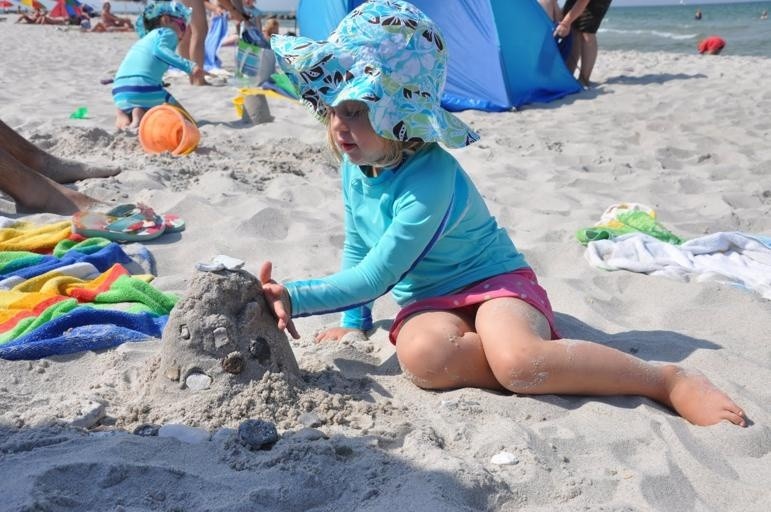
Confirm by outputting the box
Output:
[171,17,186,32]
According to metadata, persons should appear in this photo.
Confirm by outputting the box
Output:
[0,0,295,45]
[693,9,702,18]
[759,9,768,19]
[695,35,725,55]
[0,118,125,217]
[175,0,250,87]
[111,2,216,131]
[260,95,748,430]
[550,0,612,85]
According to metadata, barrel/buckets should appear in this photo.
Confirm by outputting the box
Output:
[138,102,202,158]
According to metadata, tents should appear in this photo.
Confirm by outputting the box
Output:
[296,0,584,113]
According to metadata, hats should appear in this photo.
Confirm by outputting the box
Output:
[136,1,193,38]
[270,2,480,151]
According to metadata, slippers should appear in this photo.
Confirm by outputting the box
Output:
[161,214,185,233]
[70,202,166,242]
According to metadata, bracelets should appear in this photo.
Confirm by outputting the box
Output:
[557,20,570,30]
